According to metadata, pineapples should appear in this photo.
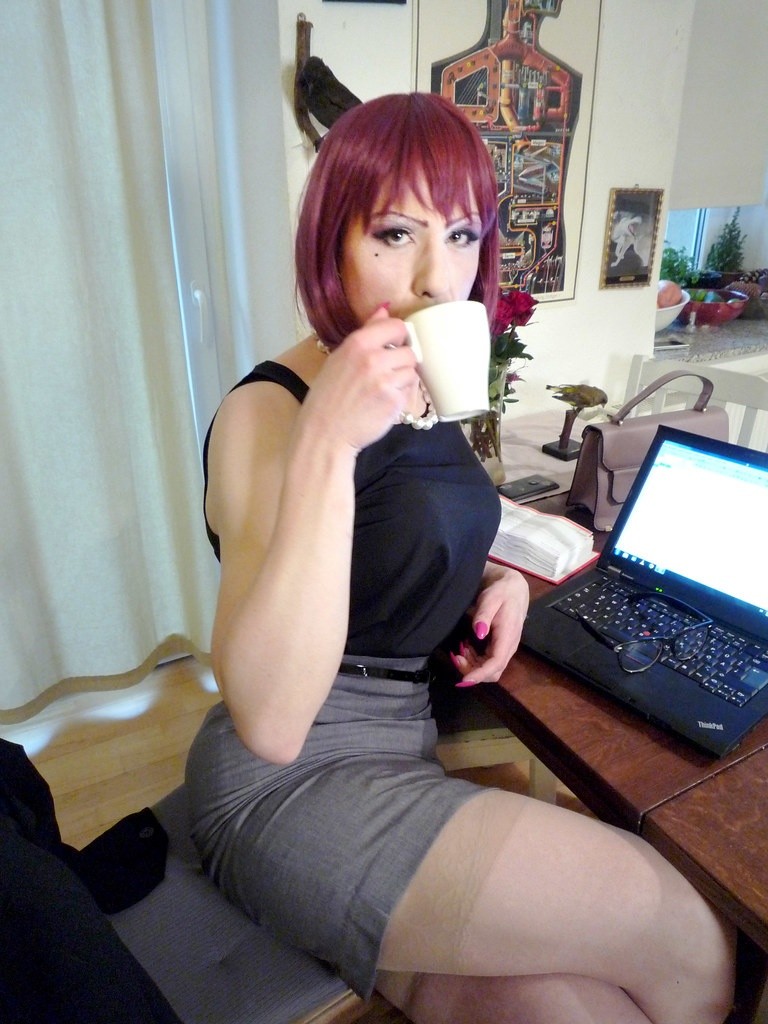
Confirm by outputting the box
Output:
[726,281,761,297]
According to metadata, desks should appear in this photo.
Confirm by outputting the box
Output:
[436,491,768,1024]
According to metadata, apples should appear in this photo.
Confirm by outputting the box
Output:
[658,279,682,308]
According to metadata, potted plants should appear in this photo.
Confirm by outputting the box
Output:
[706,206,749,289]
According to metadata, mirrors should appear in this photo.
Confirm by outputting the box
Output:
[659,204,768,289]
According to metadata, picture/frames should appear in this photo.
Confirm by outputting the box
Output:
[598,188,665,289]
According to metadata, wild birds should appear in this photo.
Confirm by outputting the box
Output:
[545,385,608,417]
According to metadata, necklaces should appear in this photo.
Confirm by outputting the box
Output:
[309,327,439,430]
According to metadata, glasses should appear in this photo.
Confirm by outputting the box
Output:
[575,592,713,674]
[572,591,714,673]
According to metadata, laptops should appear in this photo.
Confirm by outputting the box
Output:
[521,423,768,757]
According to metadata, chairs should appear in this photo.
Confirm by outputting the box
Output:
[622,355,768,452]
[430,678,559,805]
[0,739,386,1024]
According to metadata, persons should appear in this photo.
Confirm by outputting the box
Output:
[185,93,737,1024]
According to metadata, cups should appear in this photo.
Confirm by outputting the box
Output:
[387,301,490,431]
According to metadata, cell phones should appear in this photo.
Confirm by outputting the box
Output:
[495,474,560,501]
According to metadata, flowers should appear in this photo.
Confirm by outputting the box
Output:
[466,289,540,464]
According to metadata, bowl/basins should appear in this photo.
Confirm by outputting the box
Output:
[677,288,749,328]
[655,291,690,331]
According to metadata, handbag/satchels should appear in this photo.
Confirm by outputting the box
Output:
[566,370,729,532]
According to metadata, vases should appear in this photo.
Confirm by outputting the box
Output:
[460,361,510,487]
